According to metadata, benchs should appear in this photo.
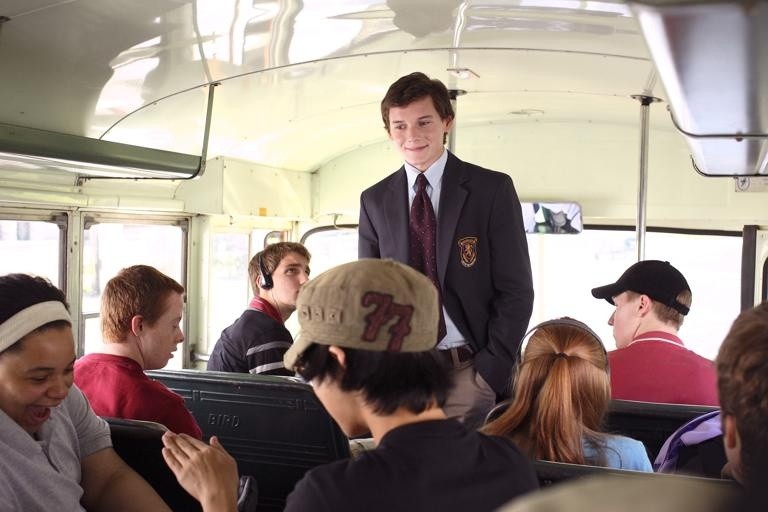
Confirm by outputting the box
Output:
[97,363,752,511]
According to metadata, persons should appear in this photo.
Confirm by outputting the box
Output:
[354,71,537,430]
[587,259,722,411]
[651,255,768,476]
[207,240,314,384]
[1,273,176,510]
[718,299,768,510]
[479,313,655,474]
[71,263,203,442]
[161,255,541,510]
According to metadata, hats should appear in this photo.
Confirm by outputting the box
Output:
[279,254,440,374]
[588,259,693,317]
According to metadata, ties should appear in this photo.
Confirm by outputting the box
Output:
[407,171,450,347]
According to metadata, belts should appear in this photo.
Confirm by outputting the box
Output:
[442,344,476,366]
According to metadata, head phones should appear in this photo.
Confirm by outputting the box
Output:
[515,319,611,383]
[259,252,273,289]
